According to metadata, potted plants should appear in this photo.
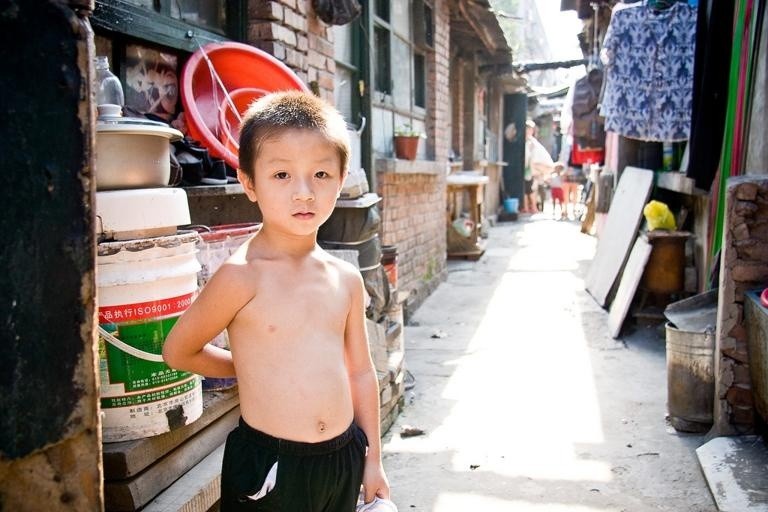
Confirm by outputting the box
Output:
[391,123,428,160]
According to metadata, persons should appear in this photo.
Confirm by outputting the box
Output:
[523,120,539,213]
[549,164,565,217]
[561,145,585,220]
[163,89,391,512]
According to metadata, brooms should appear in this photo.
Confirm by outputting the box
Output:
[447,206,480,252]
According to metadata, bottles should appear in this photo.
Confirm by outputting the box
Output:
[664,141,673,171]
[97,55,125,119]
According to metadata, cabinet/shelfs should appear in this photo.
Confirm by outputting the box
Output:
[446,173,490,260]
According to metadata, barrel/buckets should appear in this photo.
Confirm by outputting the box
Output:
[380,244,399,301]
[505,198,519,213]
[199,222,265,393]
[97,229,205,442]
[666,323,714,434]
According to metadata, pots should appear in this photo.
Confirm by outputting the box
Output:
[94,105,183,187]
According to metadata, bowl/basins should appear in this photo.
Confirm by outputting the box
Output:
[217,87,274,153]
[181,41,319,171]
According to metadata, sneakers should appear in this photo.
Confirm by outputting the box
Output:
[144,113,214,176]
[200,159,228,185]
[172,141,203,186]
[123,107,184,186]
[226,163,237,183]
